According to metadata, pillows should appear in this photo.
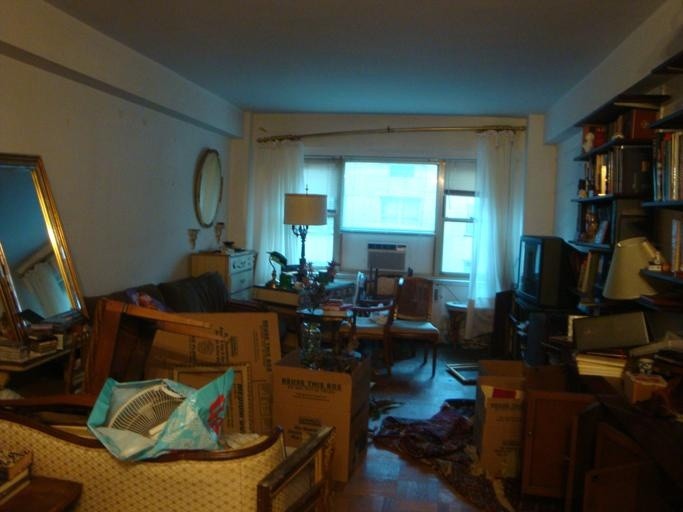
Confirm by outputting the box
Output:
[123,287,171,313]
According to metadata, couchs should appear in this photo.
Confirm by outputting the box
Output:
[86,272,295,343]
[0,409,340,512]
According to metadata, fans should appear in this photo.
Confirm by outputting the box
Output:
[100,375,193,448]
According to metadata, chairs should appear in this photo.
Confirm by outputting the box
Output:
[335,266,440,377]
[0,294,230,409]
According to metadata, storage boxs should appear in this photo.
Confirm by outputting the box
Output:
[146,314,371,488]
[471,355,539,482]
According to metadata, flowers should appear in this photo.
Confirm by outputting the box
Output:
[287,253,340,320]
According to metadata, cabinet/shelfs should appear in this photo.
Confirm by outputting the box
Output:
[633,108,682,290]
[190,248,255,301]
[566,91,670,254]
[503,294,569,367]
[523,368,682,512]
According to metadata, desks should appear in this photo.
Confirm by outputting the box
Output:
[0,472,82,512]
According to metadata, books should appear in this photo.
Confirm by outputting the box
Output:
[573,349,629,381]
[576,131,682,294]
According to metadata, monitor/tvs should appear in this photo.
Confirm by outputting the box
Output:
[517,235,587,308]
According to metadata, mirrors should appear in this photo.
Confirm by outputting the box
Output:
[0,151,89,330]
[192,147,224,229]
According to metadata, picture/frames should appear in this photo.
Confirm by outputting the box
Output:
[444,360,475,385]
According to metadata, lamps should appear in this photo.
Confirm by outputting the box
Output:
[282,181,328,275]
[264,249,288,289]
[600,237,669,308]
